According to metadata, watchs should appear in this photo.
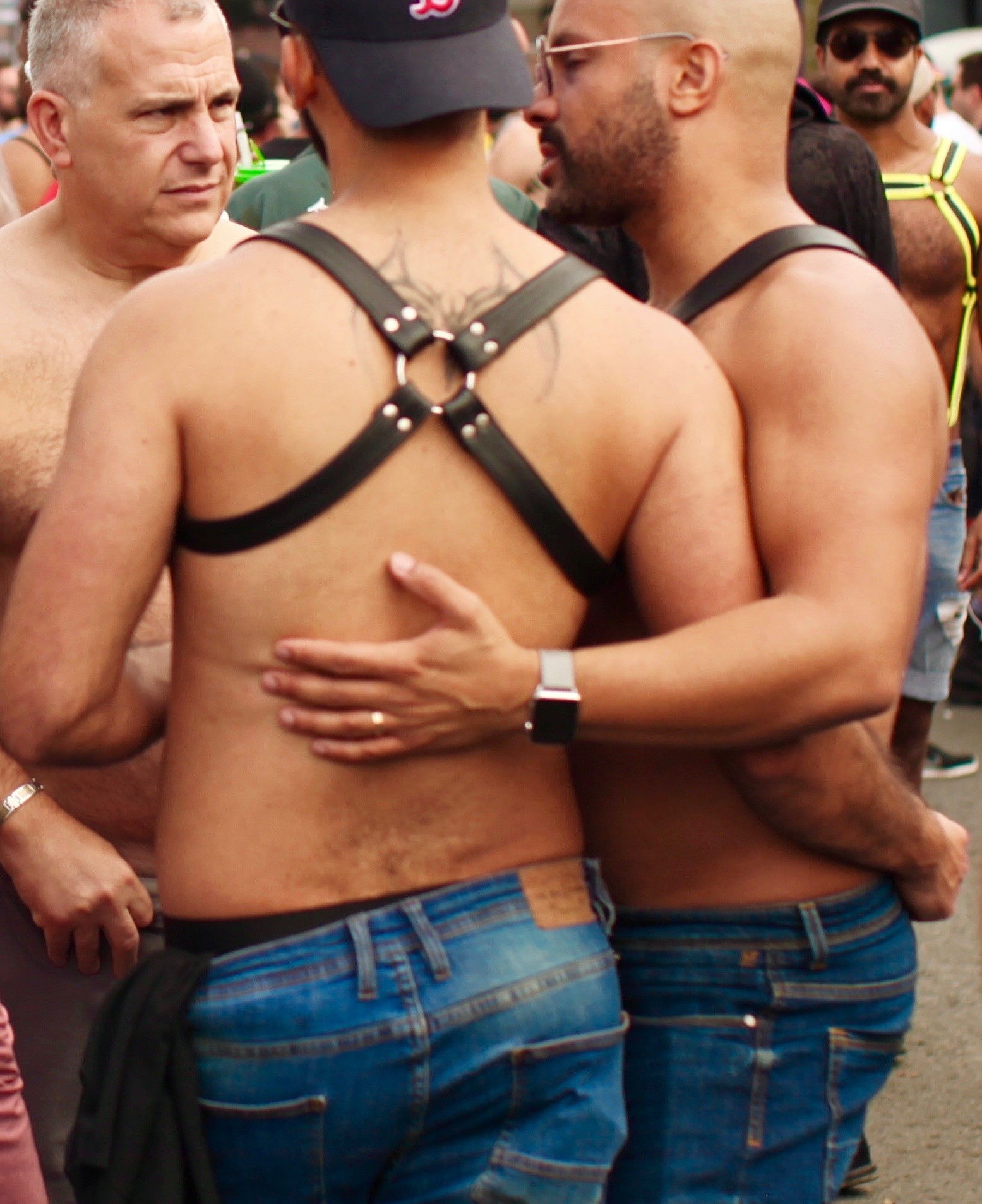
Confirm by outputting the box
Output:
[528,649,582,744]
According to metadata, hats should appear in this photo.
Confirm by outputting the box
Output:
[815,0,922,53]
[908,57,936,102]
[273,0,534,129]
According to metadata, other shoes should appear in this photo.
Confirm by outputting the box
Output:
[840,1129,878,1187]
[923,742,980,779]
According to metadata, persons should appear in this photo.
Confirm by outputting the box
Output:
[255,0,917,1204]
[0,0,982,1204]
[0,0,972,1204]
[0,0,256,1204]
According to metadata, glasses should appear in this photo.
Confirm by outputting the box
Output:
[823,29,916,62]
[534,34,727,97]
[268,0,297,40]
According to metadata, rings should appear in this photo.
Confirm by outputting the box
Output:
[371,711,385,740]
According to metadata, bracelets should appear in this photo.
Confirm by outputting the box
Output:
[0,778,44,822]
